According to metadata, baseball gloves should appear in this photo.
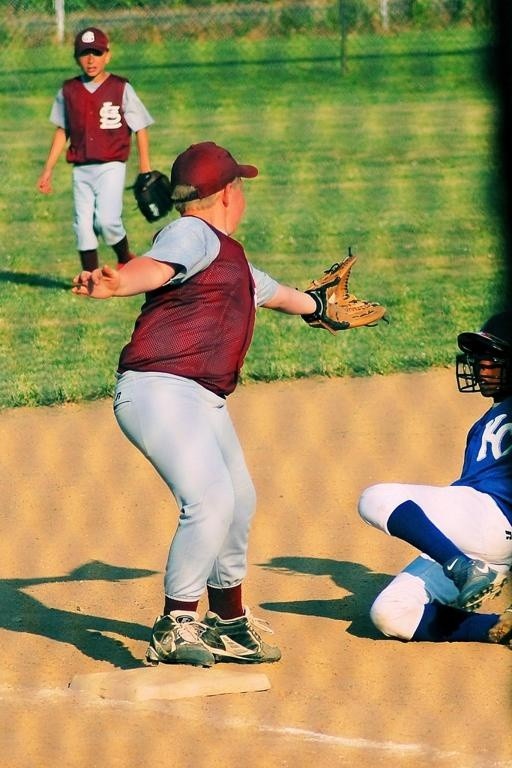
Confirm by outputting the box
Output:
[296,246,391,336]
[133,170,173,224]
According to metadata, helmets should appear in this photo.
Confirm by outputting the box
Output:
[456,311,510,355]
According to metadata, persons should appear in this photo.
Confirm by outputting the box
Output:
[34,27,157,284]
[358,311,511,653]
[72,138,389,667]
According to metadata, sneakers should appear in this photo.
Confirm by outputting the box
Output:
[488,607,512,651]
[116,253,136,271]
[193,609,282,665]
[143,609,215,669]
[442,556,511,614]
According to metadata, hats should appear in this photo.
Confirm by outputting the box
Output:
[167,140,260,201]
[74,24,110,60]
